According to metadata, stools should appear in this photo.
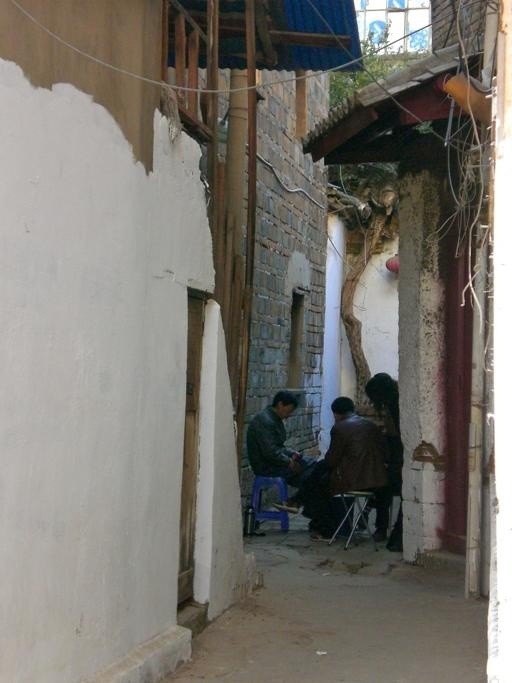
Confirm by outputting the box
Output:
[250,475,289,533]
[328,491,380,552]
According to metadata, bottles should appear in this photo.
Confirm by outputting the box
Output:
[245,506,256,535]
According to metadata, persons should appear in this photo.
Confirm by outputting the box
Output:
[246,390,320,537]
[273,395,387,540]
[366,371,402,550]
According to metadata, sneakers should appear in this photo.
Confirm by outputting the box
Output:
[371,526,388,543]
[310,533,337,542]
[272,498,301,514]
